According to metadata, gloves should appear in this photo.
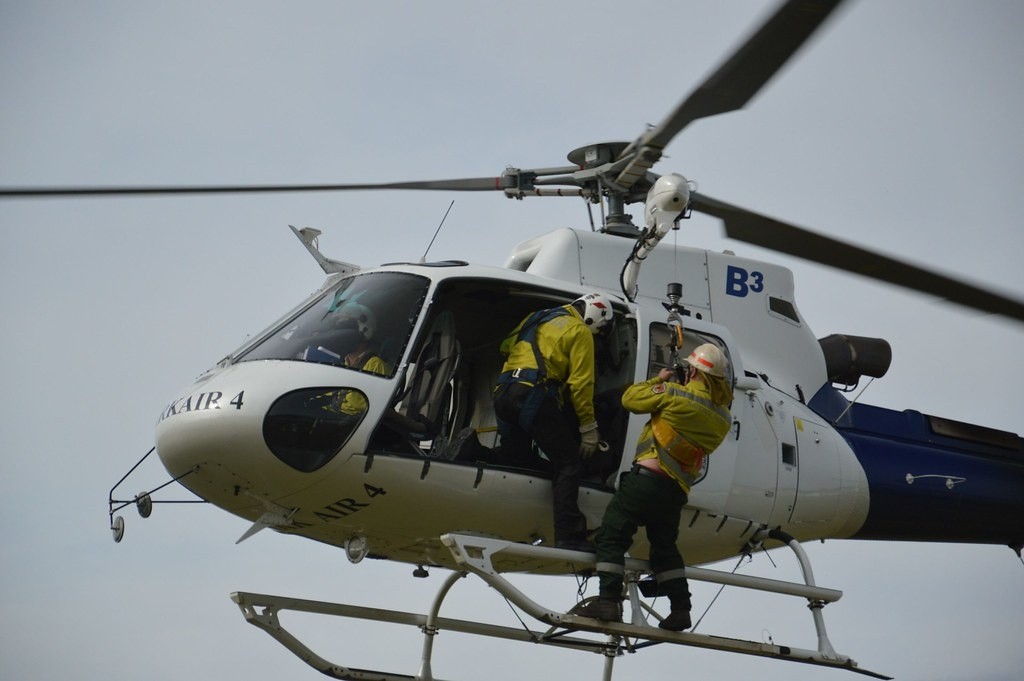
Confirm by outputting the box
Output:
[578,429,600,459]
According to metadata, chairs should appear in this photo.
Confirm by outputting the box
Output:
[383,311,458,440]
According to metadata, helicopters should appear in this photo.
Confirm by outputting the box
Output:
[0,0,1024,680]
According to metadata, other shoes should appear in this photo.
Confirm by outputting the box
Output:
[659,609,691,631]
[574,599,621,623]
[440,427,480,462]
[556,532,595,552]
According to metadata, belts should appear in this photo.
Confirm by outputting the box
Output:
[637,468,676,487]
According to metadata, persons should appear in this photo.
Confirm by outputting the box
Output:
[570,342,734,633]
[496,292,612,551]
[304,305,387,423]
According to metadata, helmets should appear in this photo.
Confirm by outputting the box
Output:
[572,293,614,334]
[682,343,726,378]
[336,304,377,341]
